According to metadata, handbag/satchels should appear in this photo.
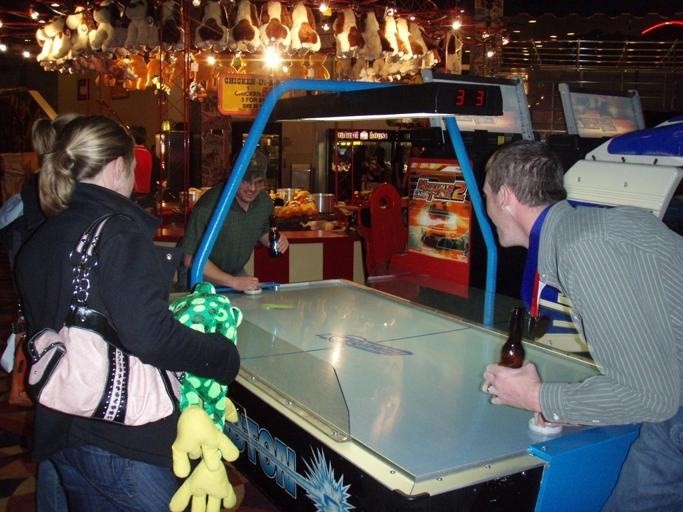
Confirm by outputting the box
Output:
[8,324,36,408]
[28,212,182,427]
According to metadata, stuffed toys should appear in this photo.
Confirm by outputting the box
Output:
[36,0,463,103]
[36,0,463,102]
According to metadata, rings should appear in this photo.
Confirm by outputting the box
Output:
[487,384,492,393]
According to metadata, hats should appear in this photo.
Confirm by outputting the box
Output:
[233,150,268,182]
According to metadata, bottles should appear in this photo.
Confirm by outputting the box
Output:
[502,306,525,368]
[269,217,283,257]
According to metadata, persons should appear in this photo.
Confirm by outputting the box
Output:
[179,150,289,292]
[482,137,683,512]
[360,146,393,191]
[2,112,242,511]
